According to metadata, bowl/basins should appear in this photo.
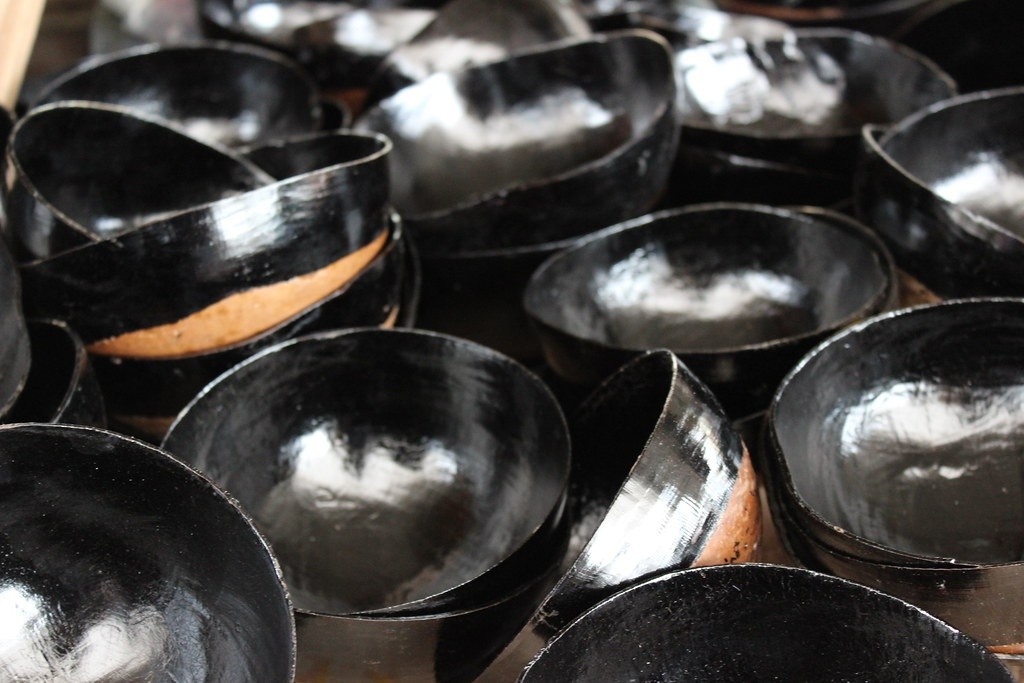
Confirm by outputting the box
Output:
[0,0,1024,683]
